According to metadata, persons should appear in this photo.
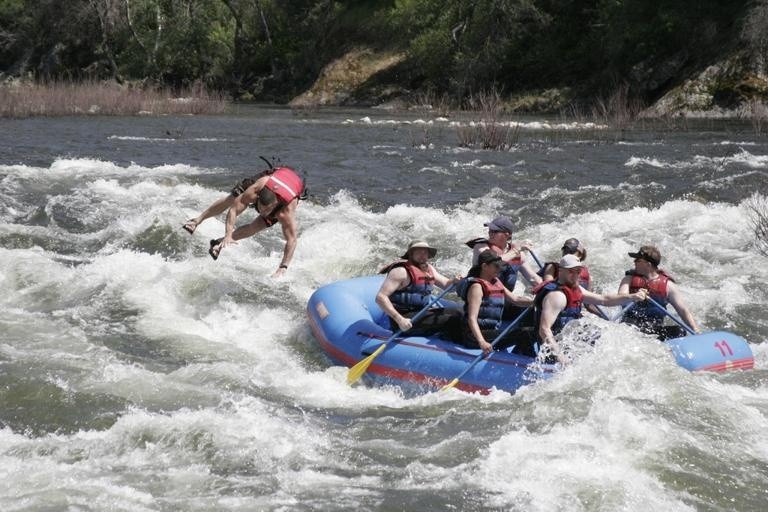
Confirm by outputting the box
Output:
[182,166,305,279]
[457,248,538,359]
[618,246,697,342]
[535,237,605,319]
[534,254,645,366]
[375,240,464,345]
[466,217,543,328]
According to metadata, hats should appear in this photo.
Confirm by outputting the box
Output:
[401,239,438,259]
[483,216,515,234]
[558,253,583,269]
[477,248,502,266]
[627,248,660,267]
[560,237,584,255]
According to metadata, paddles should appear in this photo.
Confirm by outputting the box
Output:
[348,279,459,385]
[440,302,536,391]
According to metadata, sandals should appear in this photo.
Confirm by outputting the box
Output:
[208,239,224,260]
[181,218,198,235]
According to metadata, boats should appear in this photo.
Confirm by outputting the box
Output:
[308,274,757,396]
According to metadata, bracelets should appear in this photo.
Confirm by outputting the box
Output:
[279,263,288,269]
